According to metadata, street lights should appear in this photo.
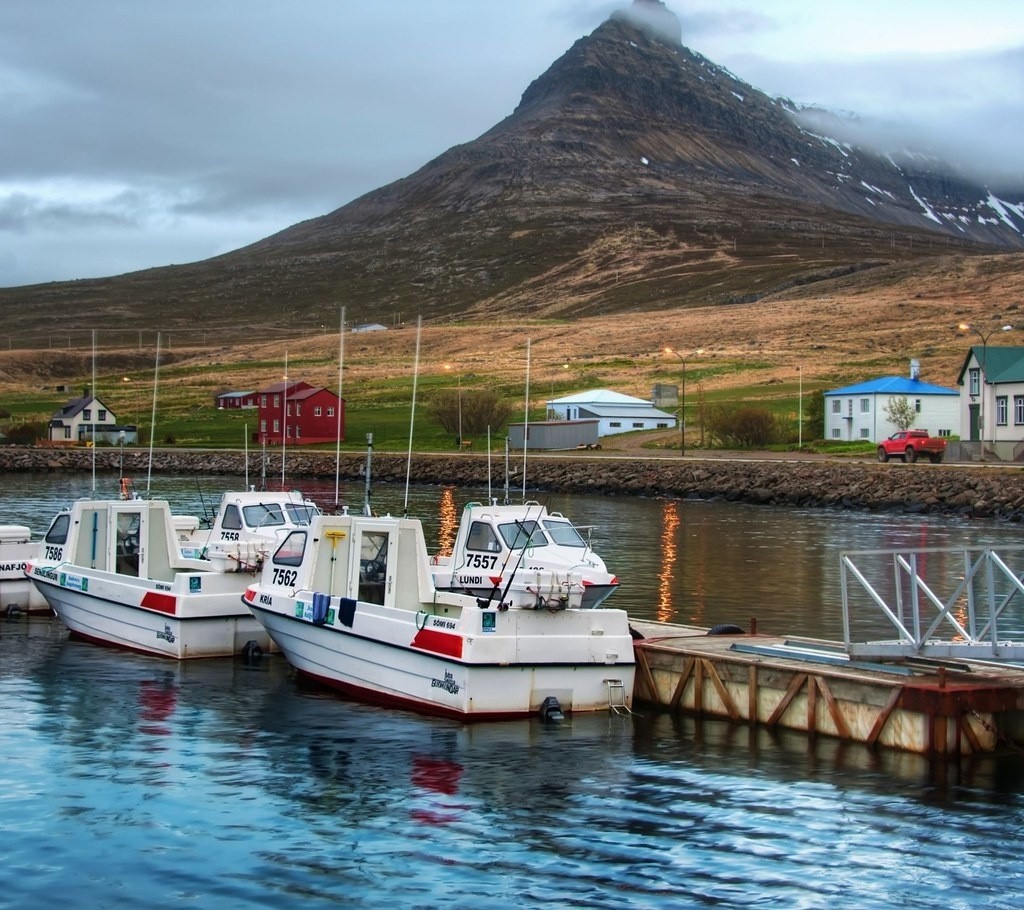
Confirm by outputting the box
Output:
[283,376,297,448]
[551,364,568,418]
[959,324,1012,461]
[664,349,705,456]
[795,365,802,446]
[123,377,138,446]
[445,365,462,450]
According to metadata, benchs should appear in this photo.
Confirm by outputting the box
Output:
[456,441,472,451]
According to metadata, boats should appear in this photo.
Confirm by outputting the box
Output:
[239,515,638,719]
[1,523,55,614]
[24,500,279,661]
[428,501,621,614]
[179,491,322,568]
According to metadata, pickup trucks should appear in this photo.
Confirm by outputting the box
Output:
[876,429,946,463]
